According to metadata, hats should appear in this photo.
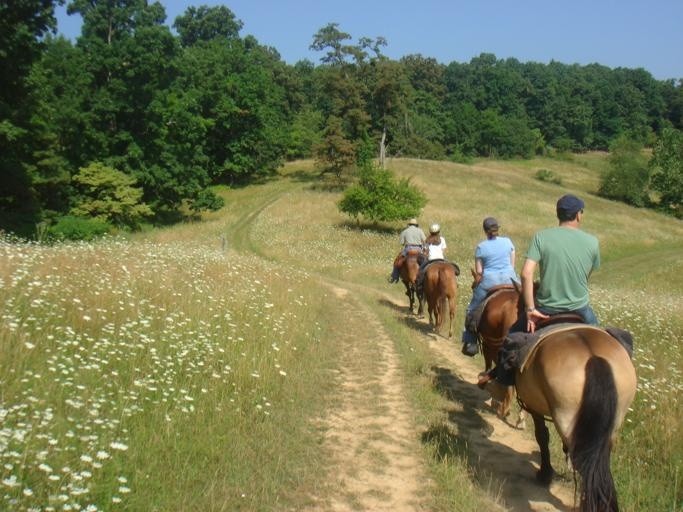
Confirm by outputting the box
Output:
[557,193,585,215]
[483,217,498,231]
[407,219,419,225]
[429,224,441,233]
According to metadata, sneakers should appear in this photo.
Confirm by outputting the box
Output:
[387,275,399,283]
[462,343,479,358]
[478,372,508,402]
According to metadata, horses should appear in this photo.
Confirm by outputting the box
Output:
[399,253,428,318]
[422,247,458,341]
[510,277,638,512]
[470,267,526,430]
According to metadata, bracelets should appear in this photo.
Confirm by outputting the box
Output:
[524,307,536,312]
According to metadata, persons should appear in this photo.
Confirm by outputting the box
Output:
[417,223,447,274]
[387,218,425,283]
[484,195,600,401]
[460,217,519,357]
